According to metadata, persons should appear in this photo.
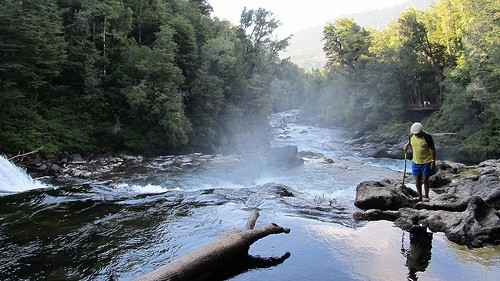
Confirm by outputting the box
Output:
[403,122,436,201]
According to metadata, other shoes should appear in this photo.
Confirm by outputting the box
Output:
[423,198,429,201]
[417,197,422,201]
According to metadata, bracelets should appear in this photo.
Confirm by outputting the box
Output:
[431,160,435,161]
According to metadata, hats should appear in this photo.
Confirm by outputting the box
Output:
[411,122,422,134]
